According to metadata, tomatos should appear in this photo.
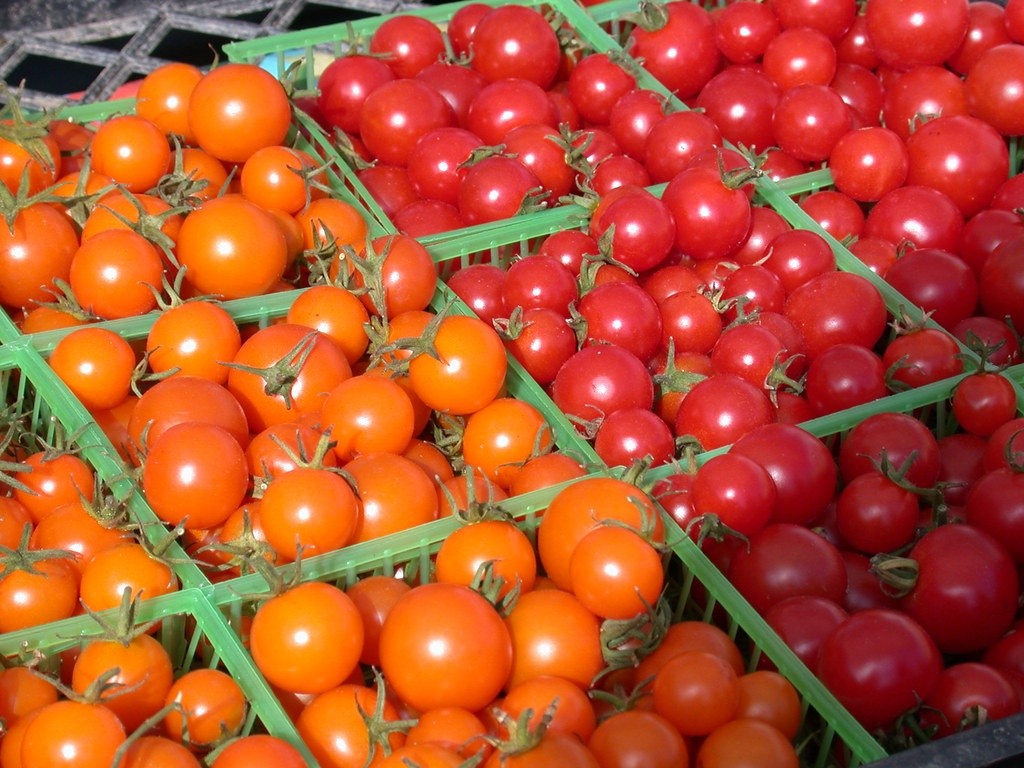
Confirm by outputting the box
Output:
[0,2,1024,768]
